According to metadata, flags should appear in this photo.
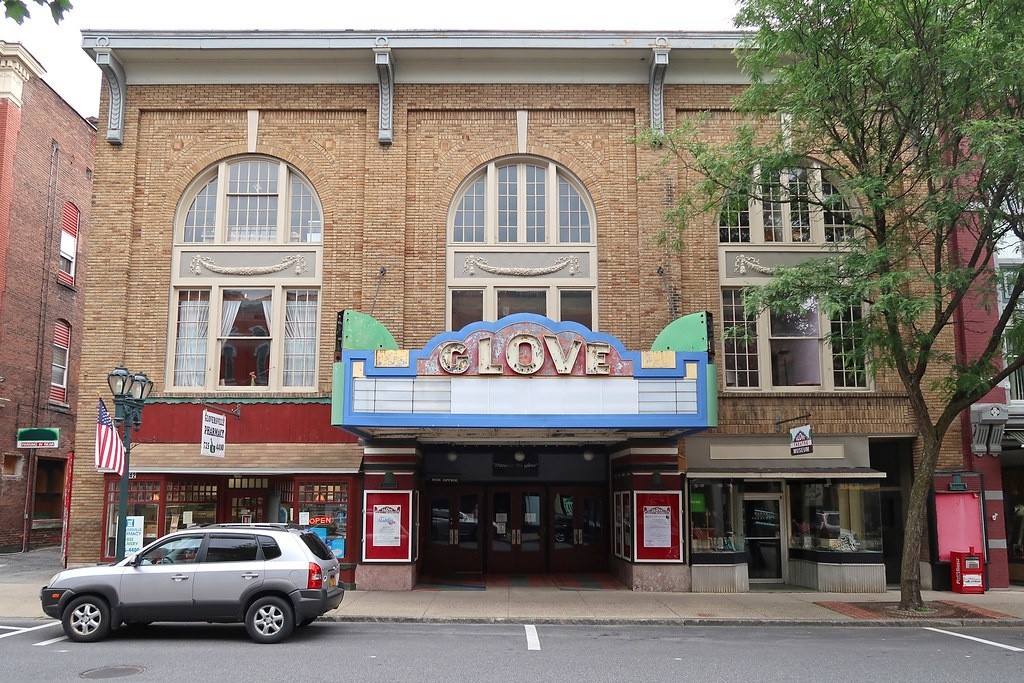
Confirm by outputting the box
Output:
[799,519,810,535]
[693,527,704,538]
[94,399,125,476]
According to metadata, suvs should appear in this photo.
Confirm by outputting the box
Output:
[39,524,344,644]
[432,507,498,541]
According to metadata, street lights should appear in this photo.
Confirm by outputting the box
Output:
[107,363,153,567]
